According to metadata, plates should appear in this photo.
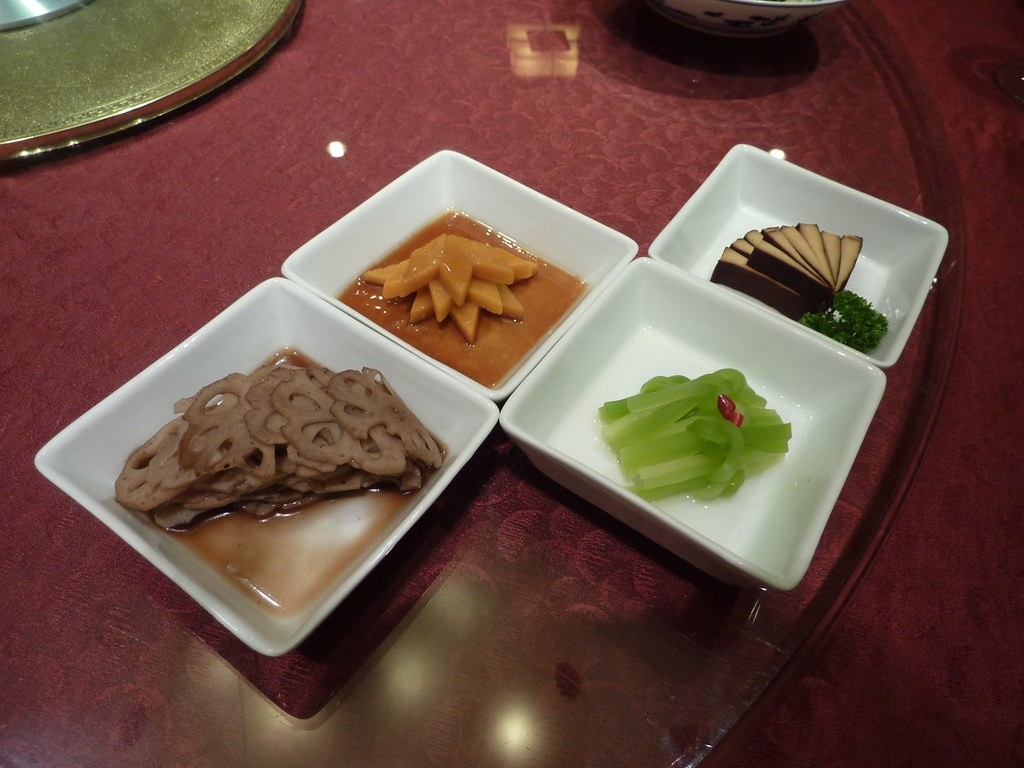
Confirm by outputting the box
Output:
[499,259,888,593]
[649,143,947,370]
[282,148,637,401]
[35,274,499,658]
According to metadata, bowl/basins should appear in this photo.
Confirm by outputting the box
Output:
[648,0,840,40]
